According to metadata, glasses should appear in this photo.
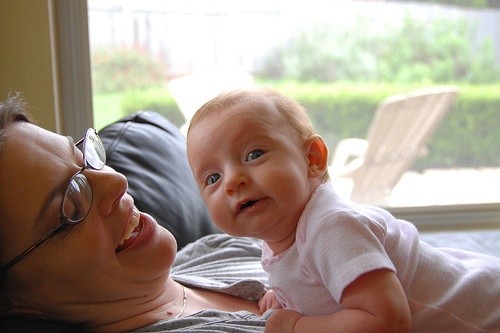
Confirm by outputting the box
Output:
[0,128,106,274]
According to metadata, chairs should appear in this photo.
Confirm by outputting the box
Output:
[326,88,456,208]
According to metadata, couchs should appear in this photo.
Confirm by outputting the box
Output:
[0,108,224,333]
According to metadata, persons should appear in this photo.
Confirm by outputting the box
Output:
[186,89,500,333]
[0,88,276,333]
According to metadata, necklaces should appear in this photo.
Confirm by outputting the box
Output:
[175,282,194,318]
